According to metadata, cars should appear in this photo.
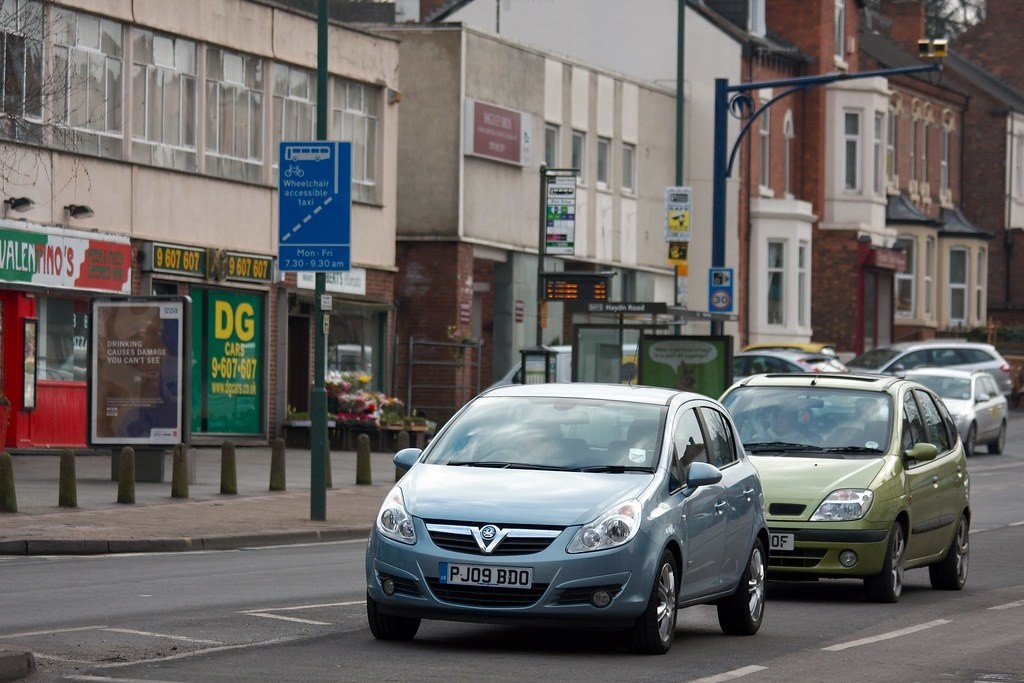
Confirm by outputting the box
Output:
[842,340,1015,412]
[328,344,373,408]
[732,351,867,418]
[868,369,1009,459]
[365,381,772,657]
[715,370,972,605]
[738,342,838,361]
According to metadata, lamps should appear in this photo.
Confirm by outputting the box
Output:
[4,197,37,213]
[64,205,95,220]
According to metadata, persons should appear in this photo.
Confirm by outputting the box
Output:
[823,398,890,441]
[751,404,815,448]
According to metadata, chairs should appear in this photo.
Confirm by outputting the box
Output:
[608,417,657,466]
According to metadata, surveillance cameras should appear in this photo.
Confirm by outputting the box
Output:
[933,39,947,57]
[917,40,929,57]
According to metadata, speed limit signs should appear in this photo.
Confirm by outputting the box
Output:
[708,287,735,314]
